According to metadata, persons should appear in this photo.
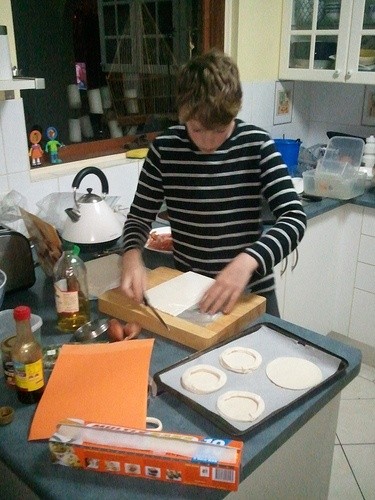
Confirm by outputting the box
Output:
[119,50,307,318]
[29,130,44,166]
[44,127,63,164]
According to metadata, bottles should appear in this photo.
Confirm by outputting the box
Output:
[53,242,91,333]
[9,307,45,403]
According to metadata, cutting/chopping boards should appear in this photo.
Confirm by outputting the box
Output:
[98,265,266,352]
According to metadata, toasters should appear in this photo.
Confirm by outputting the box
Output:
[0,223,37,294]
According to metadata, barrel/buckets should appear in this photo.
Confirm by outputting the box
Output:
[273,138,303,177]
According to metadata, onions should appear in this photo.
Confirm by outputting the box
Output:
[109,318,141,341]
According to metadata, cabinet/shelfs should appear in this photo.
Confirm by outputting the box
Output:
[273,204,356,338]
[279,0,375,85]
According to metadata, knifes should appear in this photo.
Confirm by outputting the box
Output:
[142,295,171,332]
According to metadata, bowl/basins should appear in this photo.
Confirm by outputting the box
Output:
[0,309,43,380]
[0,270,7,308]
[292,41,375,70]
[298,0,341,26]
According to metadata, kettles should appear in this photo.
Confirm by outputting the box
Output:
[61,166,128,253]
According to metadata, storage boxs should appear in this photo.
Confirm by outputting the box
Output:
[302,171,367,200]
[48,418,243,493]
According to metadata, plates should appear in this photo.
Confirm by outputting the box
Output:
[145,226,174,255]
